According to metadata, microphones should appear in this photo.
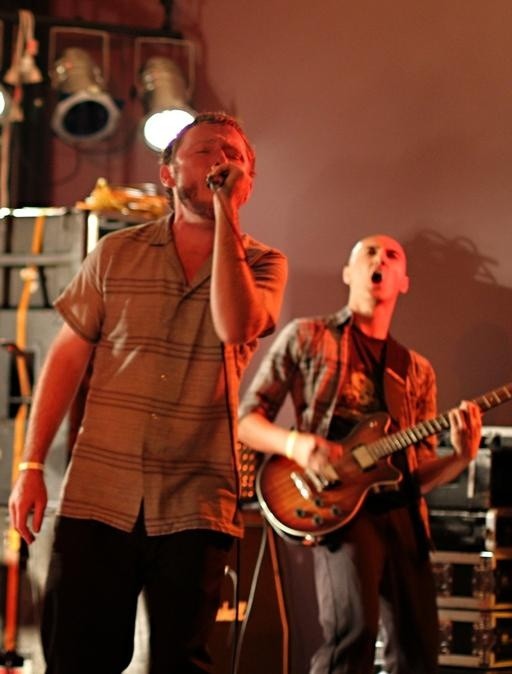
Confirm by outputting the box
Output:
[206,172,225,189]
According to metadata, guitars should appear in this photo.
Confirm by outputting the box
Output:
[254,383,510,547]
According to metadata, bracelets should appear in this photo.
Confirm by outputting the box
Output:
[285,428,300,461]
[19,461,45,471]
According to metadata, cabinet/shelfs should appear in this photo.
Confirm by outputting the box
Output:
[421,508,511,674]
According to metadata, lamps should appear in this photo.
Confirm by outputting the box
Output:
[129,37,200,154]
[48,25,125,148]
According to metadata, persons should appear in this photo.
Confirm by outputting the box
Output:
[6,107,291,673]
[236,232,486,673]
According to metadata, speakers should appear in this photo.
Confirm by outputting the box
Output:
[204,510,378,673]
